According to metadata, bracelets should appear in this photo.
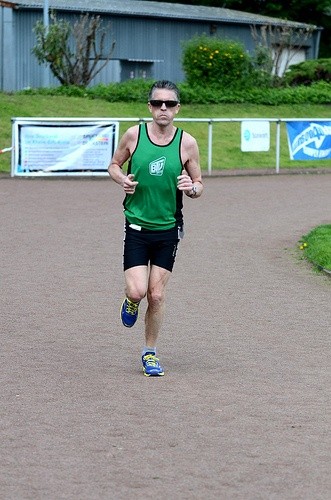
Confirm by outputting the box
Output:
[192,180,203,184]
[190,186,197,198]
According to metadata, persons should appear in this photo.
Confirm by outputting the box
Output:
[108,80,204,376]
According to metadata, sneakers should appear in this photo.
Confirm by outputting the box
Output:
[120,295,139,328]
[142,351,164,376]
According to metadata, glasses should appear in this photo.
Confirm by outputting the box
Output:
[148,100,179,107]
